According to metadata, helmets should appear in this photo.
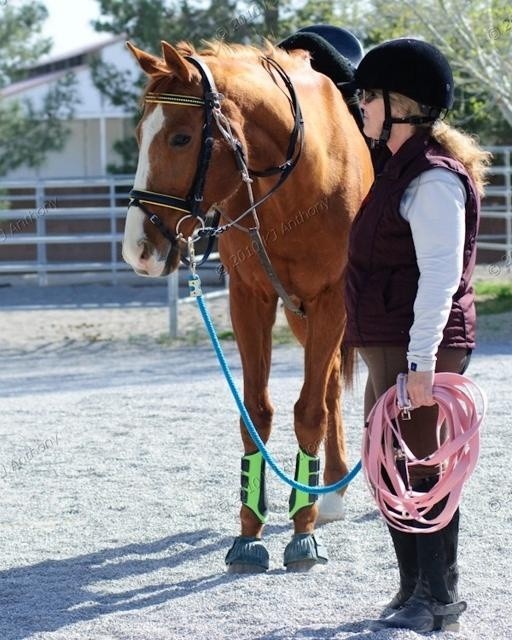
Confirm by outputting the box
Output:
[336,37,454,108]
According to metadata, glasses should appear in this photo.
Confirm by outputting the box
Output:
[351,88,379,105]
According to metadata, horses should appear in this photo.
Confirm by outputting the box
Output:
[120,30,375,574]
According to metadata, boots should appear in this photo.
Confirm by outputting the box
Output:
[362,462,461,634]
[375,459,413,609]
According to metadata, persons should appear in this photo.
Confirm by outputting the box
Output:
[340,39,495,630]
